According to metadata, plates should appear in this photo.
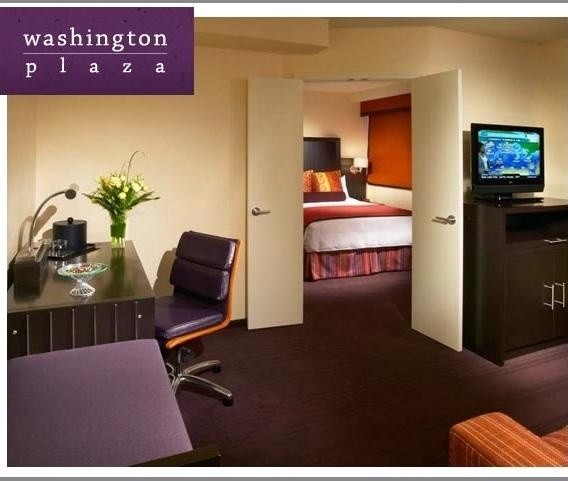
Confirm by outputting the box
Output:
[56,261,108,297]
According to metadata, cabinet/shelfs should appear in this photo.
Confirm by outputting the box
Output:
[464,197,568,367]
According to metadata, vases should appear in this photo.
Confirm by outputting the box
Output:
[110,213,126,249]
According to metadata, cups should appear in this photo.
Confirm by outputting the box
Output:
[52,240,66,257]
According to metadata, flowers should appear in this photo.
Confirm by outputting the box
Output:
[81,150,159,248]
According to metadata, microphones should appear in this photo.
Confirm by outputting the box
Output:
[28,190,76,254]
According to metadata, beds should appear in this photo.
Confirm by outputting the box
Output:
[303,170,412,282]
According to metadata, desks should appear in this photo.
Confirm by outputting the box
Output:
[7,239,155,358]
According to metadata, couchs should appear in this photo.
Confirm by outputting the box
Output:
[448,411,568,467]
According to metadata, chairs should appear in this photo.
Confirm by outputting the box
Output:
[153,231,243,406]
[7,338,220,467]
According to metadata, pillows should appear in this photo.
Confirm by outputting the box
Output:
[303,170,343,192]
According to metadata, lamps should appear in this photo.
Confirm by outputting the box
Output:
[353,158,368,175]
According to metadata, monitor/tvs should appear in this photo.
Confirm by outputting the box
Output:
[470,123,544,203]
